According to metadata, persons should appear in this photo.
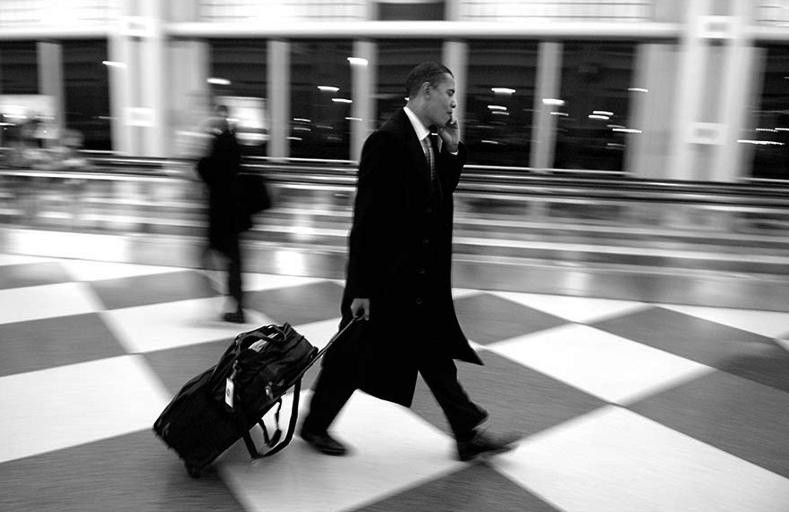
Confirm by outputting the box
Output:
[297,58,521,464]
[195,115,255,323]
[213,104,238,135]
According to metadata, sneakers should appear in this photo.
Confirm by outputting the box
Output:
[224,311,245,323]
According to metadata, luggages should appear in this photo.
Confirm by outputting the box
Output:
[153,309,364,479]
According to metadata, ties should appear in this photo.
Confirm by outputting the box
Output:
[425,136,436,179]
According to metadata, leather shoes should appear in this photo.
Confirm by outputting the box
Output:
[457,426,519,461]
[301,428,345,455]
